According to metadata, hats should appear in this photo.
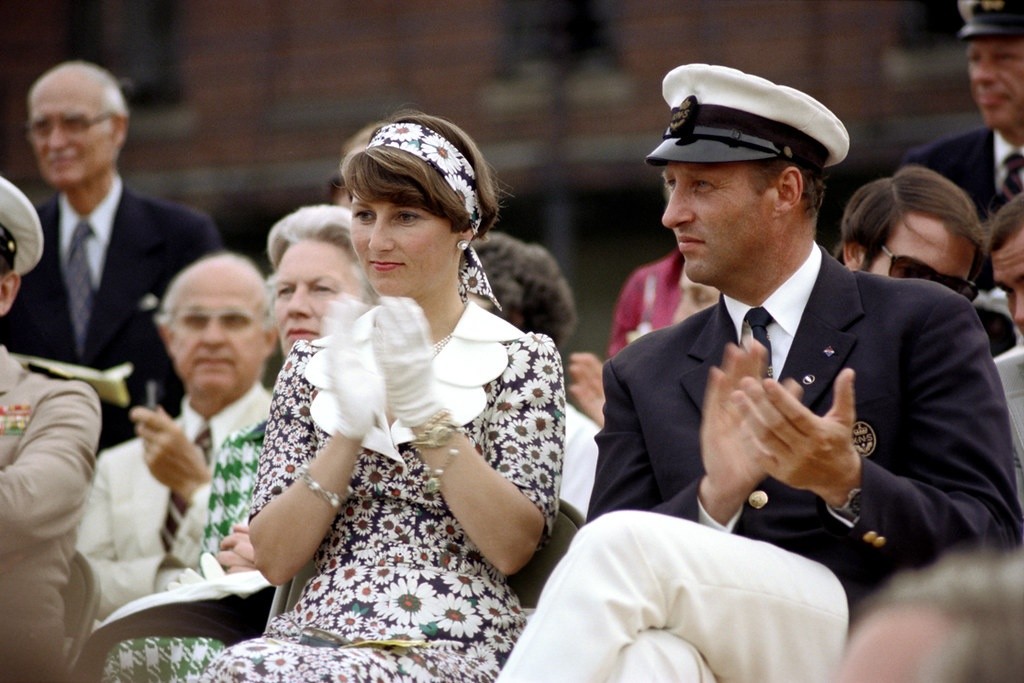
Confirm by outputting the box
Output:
[956,0,1024,39]
[644,63,851,175]
[0,176,44,281]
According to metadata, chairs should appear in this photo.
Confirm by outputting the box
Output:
[52,545,101,683]
[262,496,585,638]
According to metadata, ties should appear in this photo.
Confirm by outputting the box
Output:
[744,303,776,380]
[996,153,1024,213]
[61,220,97,358]
[158,427,213,555]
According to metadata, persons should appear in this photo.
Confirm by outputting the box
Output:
[320,167,369,213]
[495,62,1024,683]
[569,175,733,531]
[198,112,566,683]
[832,548,1024,683]
[460,232,603,613]
[0,177,103,683]
[840,166,985,336]
[102,204,380,683]
[900,0,1024,221]
[80,251,278,635]
[0,61,227,459]
[989,191,1024,523]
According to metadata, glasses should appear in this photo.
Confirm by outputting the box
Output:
[28,107,116,146]
[881,245,981,301]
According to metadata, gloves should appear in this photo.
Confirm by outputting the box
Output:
[372,294,440,430]
[321,293,384,438]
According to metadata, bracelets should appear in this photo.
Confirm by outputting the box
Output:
[426,440,470,491]
[412,409,460,449]
[296,464,342,508]
